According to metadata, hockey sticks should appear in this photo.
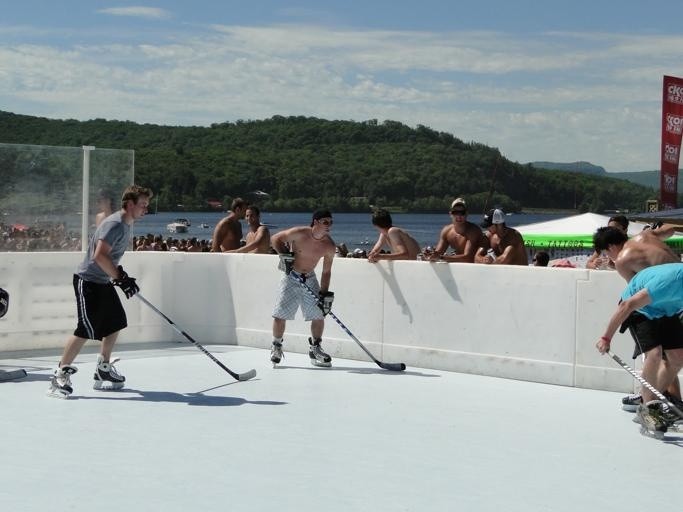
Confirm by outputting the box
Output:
[135,291,256,381]
[291,268,406,370]
[0,370,28,381]
[607,349,683,443]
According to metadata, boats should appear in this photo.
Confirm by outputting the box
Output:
[165,217,212,234]
[614,208,629,214]
[260,222,278,229]
[367,202,413,214]
[504,211,514,216]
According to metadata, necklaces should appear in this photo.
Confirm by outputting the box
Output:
[311,229,324,240]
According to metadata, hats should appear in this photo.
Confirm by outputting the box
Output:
[450,198,466,208]
[479,208,505,227]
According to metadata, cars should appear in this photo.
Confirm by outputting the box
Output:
[176,204,184,207]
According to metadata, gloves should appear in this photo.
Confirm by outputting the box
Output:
[112,263,140,299]
[277,251,294,275]
[316,291,333,317]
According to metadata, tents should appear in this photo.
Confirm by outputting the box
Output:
[512,211,648,248]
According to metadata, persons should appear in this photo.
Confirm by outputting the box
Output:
[533,215,630,269]
[272,208,336,363]
[593,224,680,404]
[367,197,528,265]
[336,243,367,259]
[52,185,154,393]
[133,198,278,255]
[1,222,81,251]
[596,263,683,432]
[96,188,117,228]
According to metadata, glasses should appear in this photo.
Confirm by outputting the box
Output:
[319,221,333,225]
[450,210,465,216]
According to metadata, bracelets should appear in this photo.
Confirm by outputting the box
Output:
[600,337,611,342]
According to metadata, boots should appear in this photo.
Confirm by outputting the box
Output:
[50,362,78,394]
[638,400,667,432]
[620,391,643,406]
[92,355,125,383]
[270,339,283,364]
[307,337,330,362]
[660,390,682,423]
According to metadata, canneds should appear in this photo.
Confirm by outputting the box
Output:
[487,248,496,262]
